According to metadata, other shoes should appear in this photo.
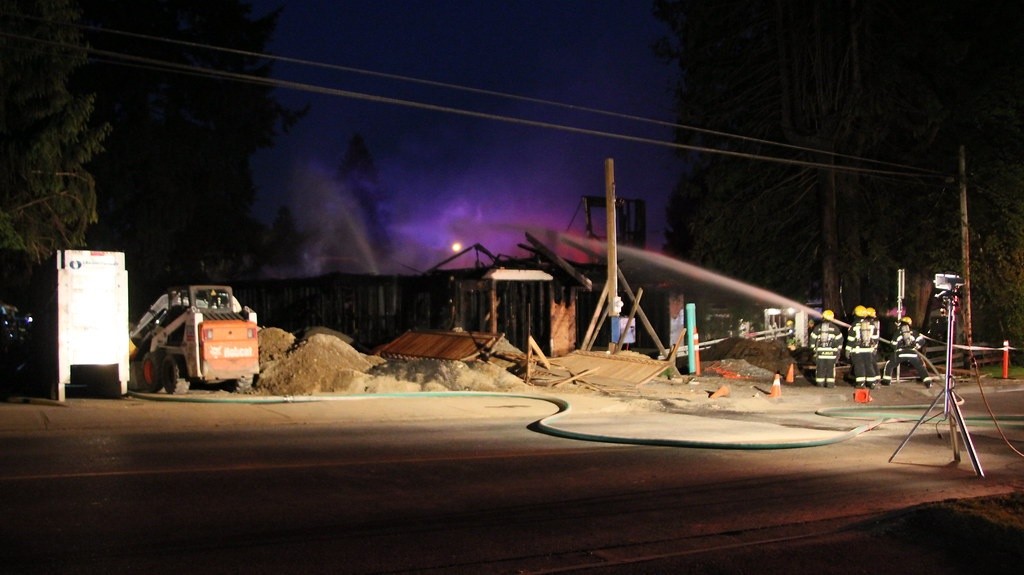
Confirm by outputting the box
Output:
[854,381,877,389]
[925,381,934,389]
[816,382,835,389]
[881,379,891,386]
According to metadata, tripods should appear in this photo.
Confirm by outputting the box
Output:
[888,290,986,481]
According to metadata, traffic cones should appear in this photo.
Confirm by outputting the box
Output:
[854,388,873,403]
[708,384,731,398]
[770,374,782,398]
[784,362,795,383]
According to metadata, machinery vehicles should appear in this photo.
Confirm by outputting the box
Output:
[130,284,260,395]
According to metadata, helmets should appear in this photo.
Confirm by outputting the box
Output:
[823,309,834,318]
[786,320,794,326]
[855,305,877,318]
[900,316,912,327]
[809,319,814,324]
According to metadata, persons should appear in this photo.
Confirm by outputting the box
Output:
[867,307,880,384]
[808,319,814,334]
[786,320,795,340]
[809,310,843,388]
[881,317,933,388]
[845,305,877,389]
